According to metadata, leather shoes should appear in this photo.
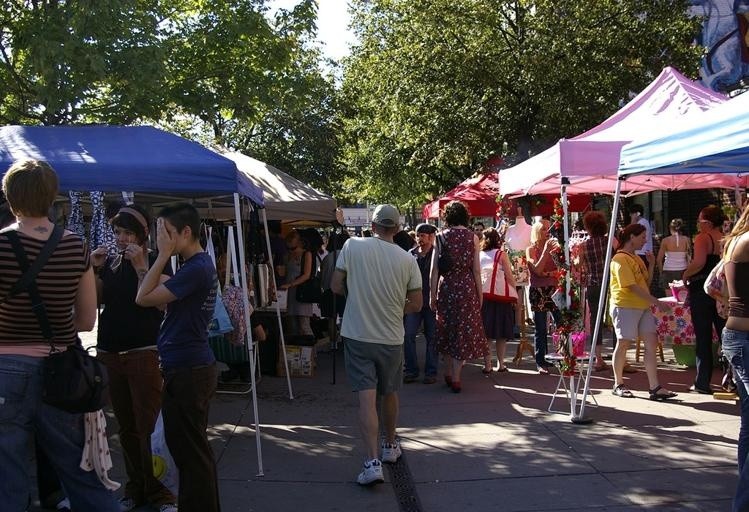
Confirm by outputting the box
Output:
[403,376,417,383]
[423,376,436,384]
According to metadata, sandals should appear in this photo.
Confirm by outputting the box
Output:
[611,384,633,398]
[649,385,677,400]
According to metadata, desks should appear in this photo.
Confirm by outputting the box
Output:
[546,353,598,416]
[635,296,719,364]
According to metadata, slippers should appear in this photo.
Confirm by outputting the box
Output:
[623,365,638,373]
[595,362,611,372]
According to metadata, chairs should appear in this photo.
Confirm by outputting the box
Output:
[206,318,263,397]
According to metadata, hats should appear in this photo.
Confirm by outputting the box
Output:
[371,203,400,228]
[416,223,439,234]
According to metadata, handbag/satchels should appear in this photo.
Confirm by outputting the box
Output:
[434,234,454,275]
[294,251,322,304]
[49,346,107,416]
[204,226,233,338]
[703,244,729,306]
[481,250,519,305]
[223,224,253,346]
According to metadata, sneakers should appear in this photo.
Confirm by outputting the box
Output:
[116,496,136,511]
[445,377,452,386]
[537,366,551,376]
[381,435,402,464]
[357,459,384,486]
[160,504,178,512]
[452,382,461,392]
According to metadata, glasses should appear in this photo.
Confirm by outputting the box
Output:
[289,246,297,251]
[104,232,132,256]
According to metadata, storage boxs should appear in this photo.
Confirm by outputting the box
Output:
[276,334,316,380]
